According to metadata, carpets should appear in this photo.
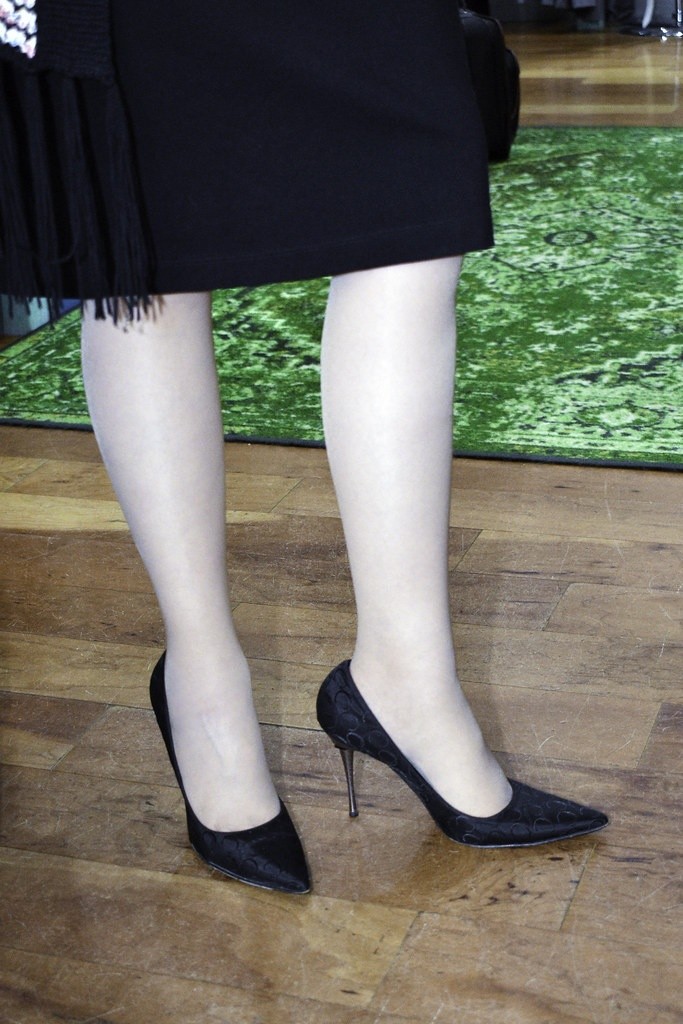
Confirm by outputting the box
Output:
[0,126,683,471]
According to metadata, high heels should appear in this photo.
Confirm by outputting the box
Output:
[315,657,610,849]
[150,643,311,894]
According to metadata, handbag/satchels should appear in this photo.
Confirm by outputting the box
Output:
[458,9,521,161]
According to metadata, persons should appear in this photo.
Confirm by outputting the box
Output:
[0,1,610,894]
